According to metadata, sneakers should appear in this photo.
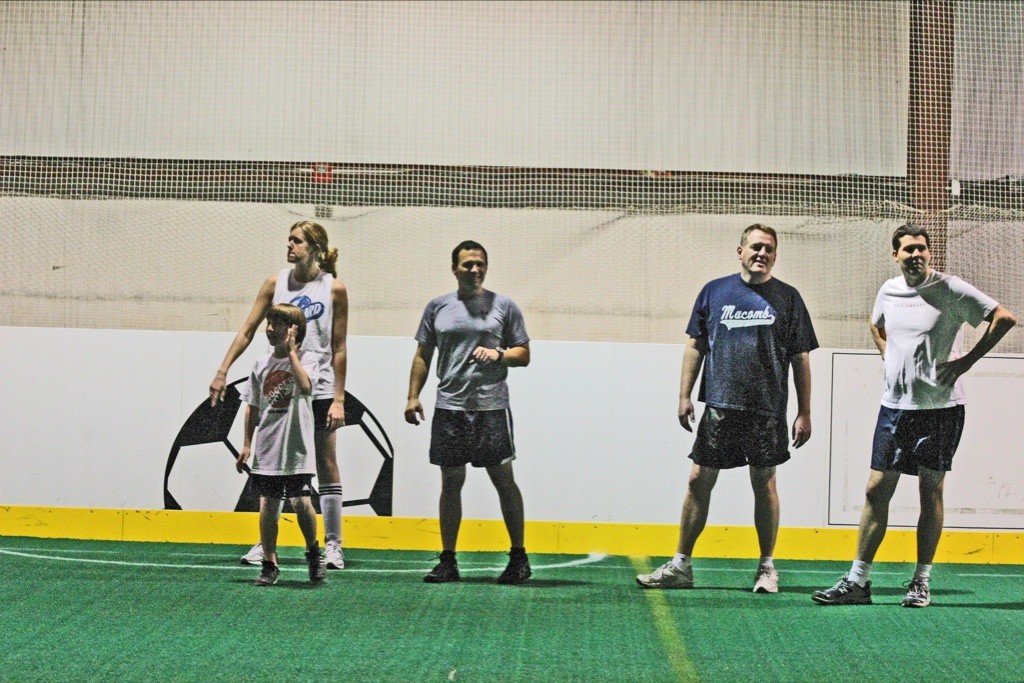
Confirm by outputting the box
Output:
[325,540,345,570]
[810,574,873,604]
[636,564,694,588]
[424,550,462,582]
[240,542,264,565]
[256,561,280,586]
[752,563,779,593]
[304,541,328,581]
[498,550,532,584]
[899,575,932,606]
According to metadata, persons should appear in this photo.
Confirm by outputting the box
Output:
[636,224,819,595]
[235,302,328,587]
[811,224,1016,607]
[208,220,349,571]
[404,241,532,585]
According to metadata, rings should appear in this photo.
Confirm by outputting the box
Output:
[951,371,956,378]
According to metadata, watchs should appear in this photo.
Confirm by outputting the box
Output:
[495,346,504,366]
